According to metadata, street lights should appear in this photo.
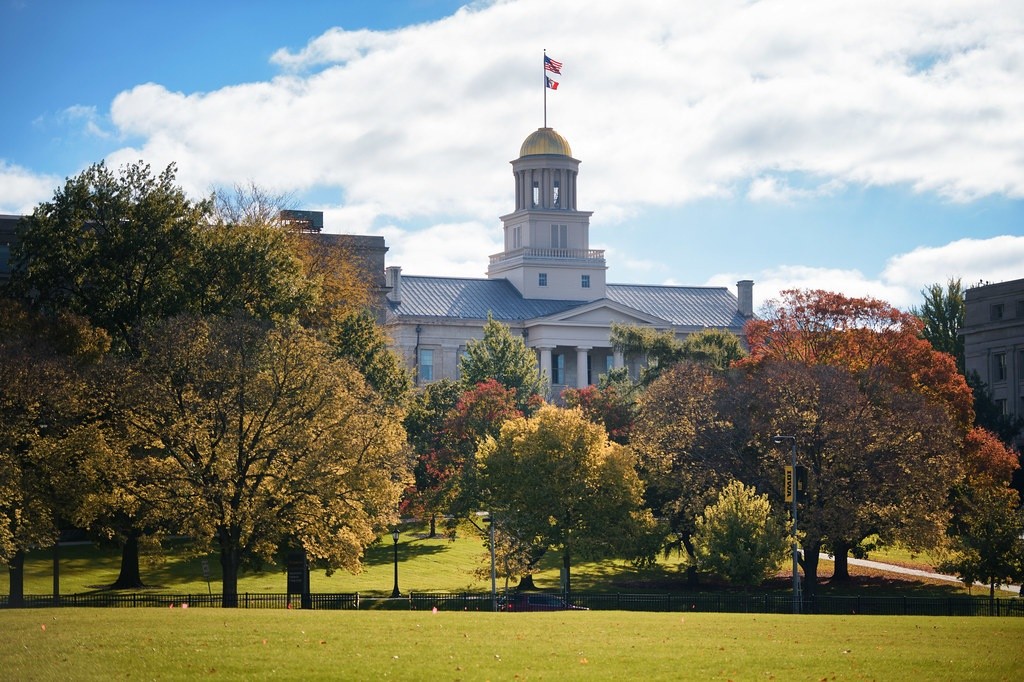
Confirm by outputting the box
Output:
[390,526,404,597]
[771,434,802,613]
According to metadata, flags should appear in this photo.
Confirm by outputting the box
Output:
[544,56,562,74]
[545,77,559,90]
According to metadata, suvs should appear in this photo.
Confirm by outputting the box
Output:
[496,590,589,612]
